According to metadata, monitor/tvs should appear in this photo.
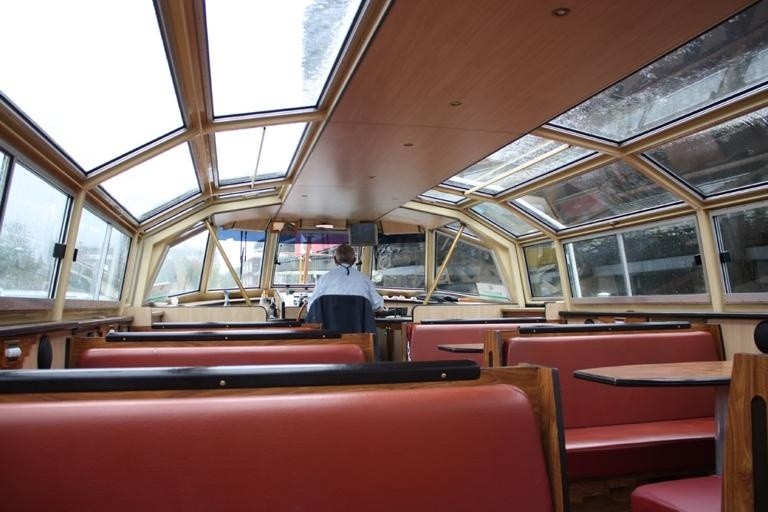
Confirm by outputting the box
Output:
[348,222,379,246]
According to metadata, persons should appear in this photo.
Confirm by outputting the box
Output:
[305,242,386,314]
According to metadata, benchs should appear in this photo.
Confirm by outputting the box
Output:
[0,318,768,512]
